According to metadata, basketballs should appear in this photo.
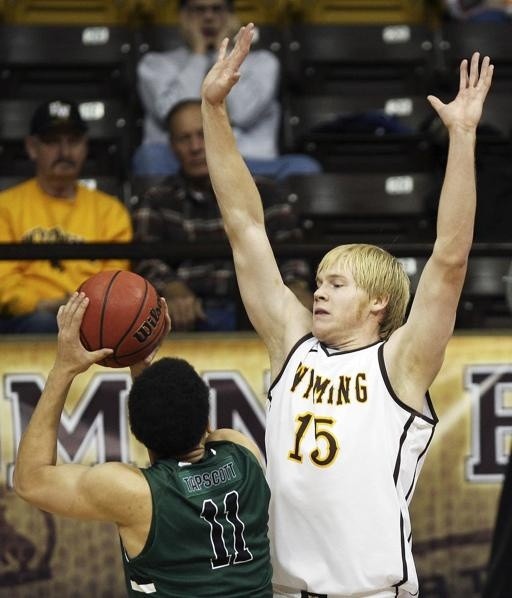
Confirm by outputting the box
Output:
[76,270,165,368]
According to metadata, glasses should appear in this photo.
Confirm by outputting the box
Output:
[189,4,226,15]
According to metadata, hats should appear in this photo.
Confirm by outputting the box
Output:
[29,102,87,139]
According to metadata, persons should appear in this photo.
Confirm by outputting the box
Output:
[200,22,495,598]
[133,99,313,331]
[13,292,274,598]
[0,100,133,333]
[132,0,322,182]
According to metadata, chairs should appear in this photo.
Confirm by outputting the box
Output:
[1,0,511,327]
